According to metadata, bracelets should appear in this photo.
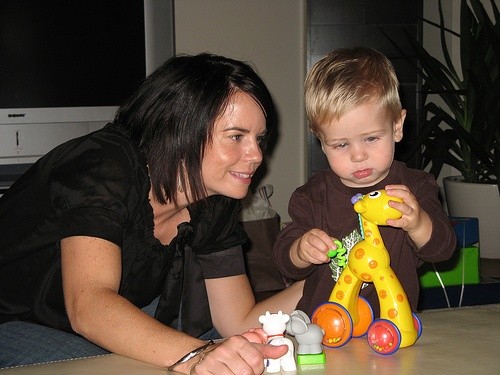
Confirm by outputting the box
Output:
[167,339,214,371]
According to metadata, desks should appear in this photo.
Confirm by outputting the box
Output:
[0,304,500,375]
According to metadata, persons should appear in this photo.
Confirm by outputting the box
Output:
[1,53,306,375]
[273,46,457,320]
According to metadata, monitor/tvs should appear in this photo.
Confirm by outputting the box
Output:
[0,0,175,123]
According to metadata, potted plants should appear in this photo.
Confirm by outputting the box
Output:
[381,0,500,258]
